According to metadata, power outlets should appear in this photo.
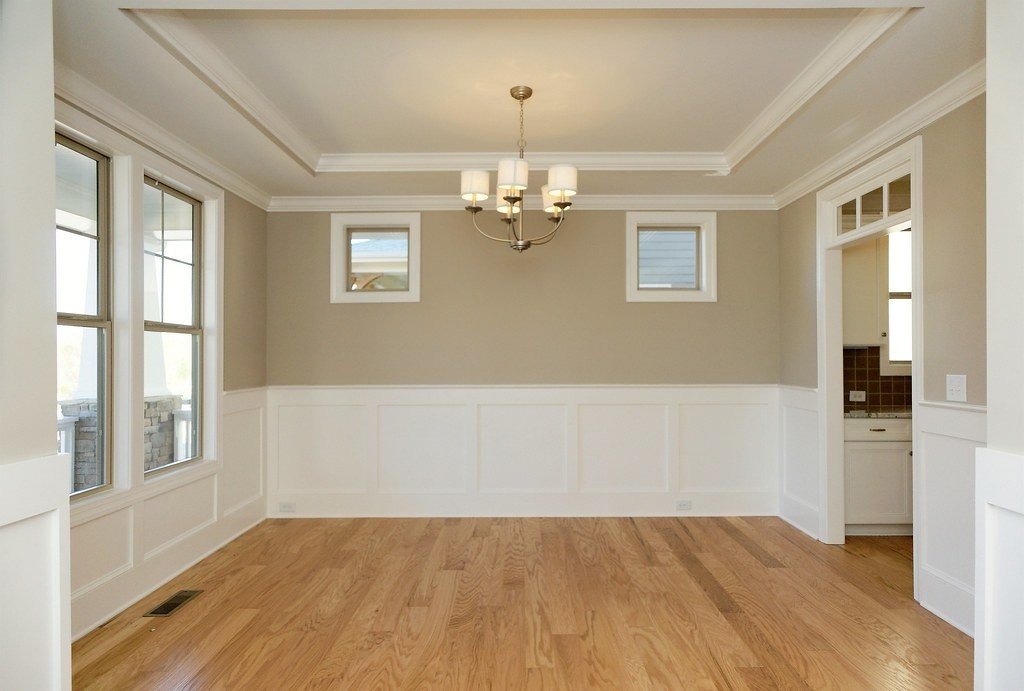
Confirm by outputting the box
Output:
[946,375,966,402]
[850,391,866,402]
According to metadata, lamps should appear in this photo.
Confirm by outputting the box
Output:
[460,86,578,254]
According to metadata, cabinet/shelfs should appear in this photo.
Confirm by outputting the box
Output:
[843,214,887,350]
[844,418,912,536]
